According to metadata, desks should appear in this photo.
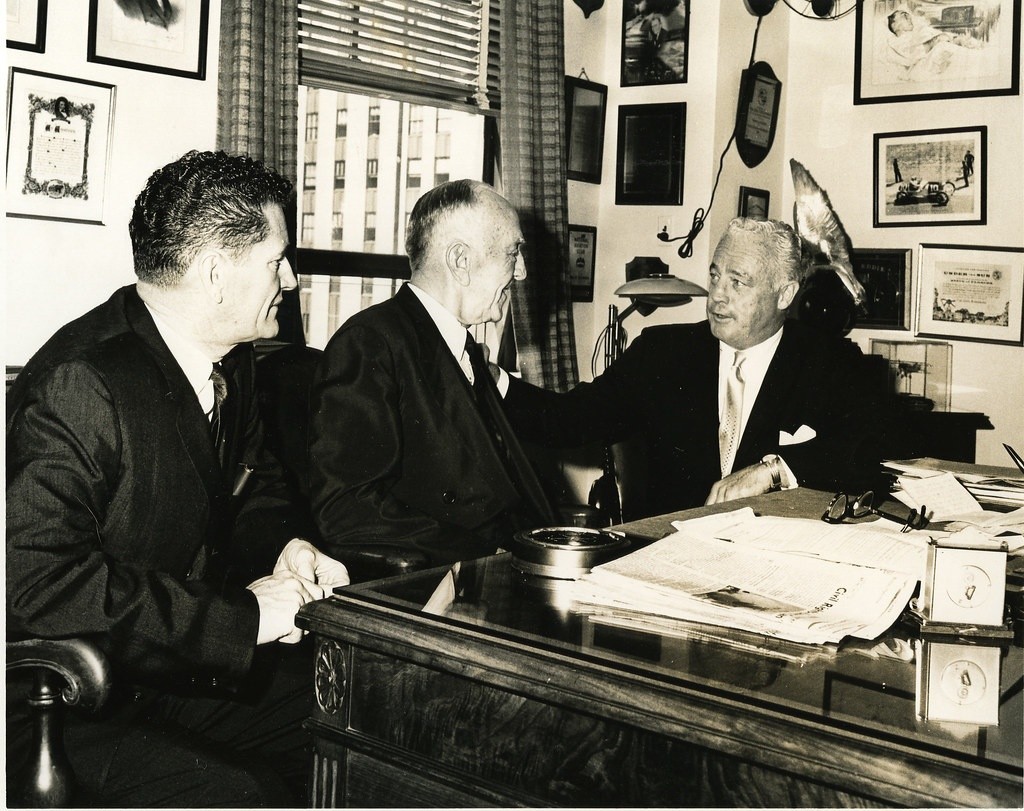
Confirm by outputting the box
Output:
[295,459,1024,808]
[885,409,993,463]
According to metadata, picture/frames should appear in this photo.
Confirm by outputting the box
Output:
[86,0,210,81]
[615,101,687,206]
[620,0,690,88]
[854,1,1022,105]
[0,1,48,54]
[3,66,117,227]
[568,224,597,303]
[850,248,912,332]
[738,185,770,221]
[872,125,988,228]
[564,75,608,185]
[914,243,1024,347]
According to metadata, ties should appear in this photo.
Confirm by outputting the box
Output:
[465,332,488,410]
[209,363,239,454]
[719,351,745,478]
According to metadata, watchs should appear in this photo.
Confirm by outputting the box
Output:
[760,453,784,492]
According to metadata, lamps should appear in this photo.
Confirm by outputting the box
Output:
[609,272,708,365]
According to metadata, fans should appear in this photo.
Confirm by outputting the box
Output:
[742,0,863,20]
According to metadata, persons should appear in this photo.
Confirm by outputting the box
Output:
[639,16,673,84]
[960,149,974,187]
[7,152,351,809]
[479,216,897,523]
[910,175,921,191]
[886,9,974,71]
[892,157,903,185]
[309,181,569,583]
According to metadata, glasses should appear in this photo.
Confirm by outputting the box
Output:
[822,489,926,532]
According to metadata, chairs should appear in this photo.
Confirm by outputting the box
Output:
[6,340,938,810]
